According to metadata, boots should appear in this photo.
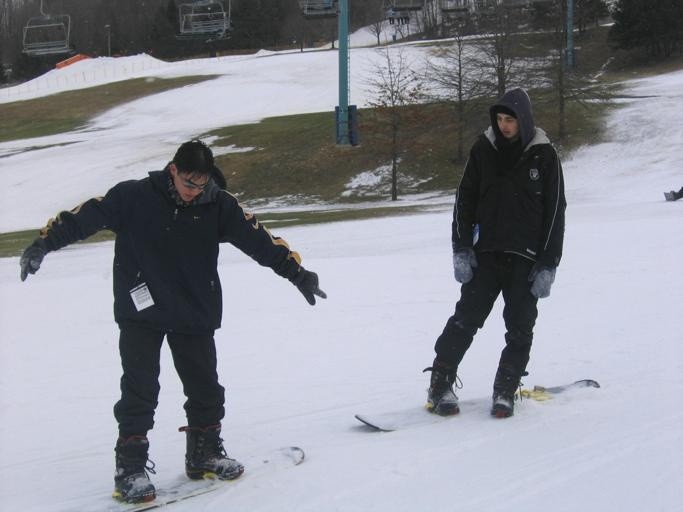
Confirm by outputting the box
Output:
[490,361,529,418]
[422,356,464,415]
[179,420,244,481]
[113,432,157,502]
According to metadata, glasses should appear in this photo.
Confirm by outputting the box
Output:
[177,170,209,191]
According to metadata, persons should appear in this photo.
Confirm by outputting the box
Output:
[388,7,409,25]
[20,139,326,504]
[428,87,566,419]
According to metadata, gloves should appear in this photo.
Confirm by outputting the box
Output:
[18,237,46,283]
[528,263,557,299]
[452,245,477,284]
[293,266,328,305]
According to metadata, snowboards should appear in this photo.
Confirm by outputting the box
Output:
[355,379,600,432]
[70,444,306,512]
